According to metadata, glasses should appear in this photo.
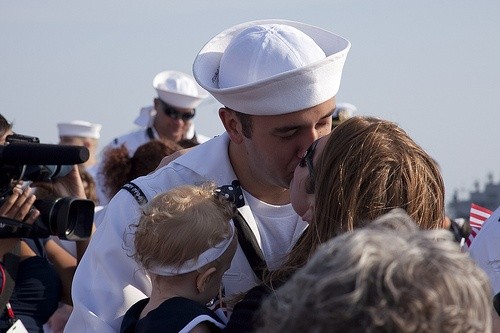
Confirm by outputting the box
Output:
[299,137,325,190]
[160,100,195,121]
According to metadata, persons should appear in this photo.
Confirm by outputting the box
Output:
[120,185,239,333]
[61,18,352,332]
[221,114,448,332]
[91,70,220,204]
[252,209,500,333]
[0,114,96,333]
[55,120,102,196]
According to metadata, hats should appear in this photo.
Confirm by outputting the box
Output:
[152,71,209,108]
[192,19,351,116]
[332,103,356,125]
[57,121,100,139]
